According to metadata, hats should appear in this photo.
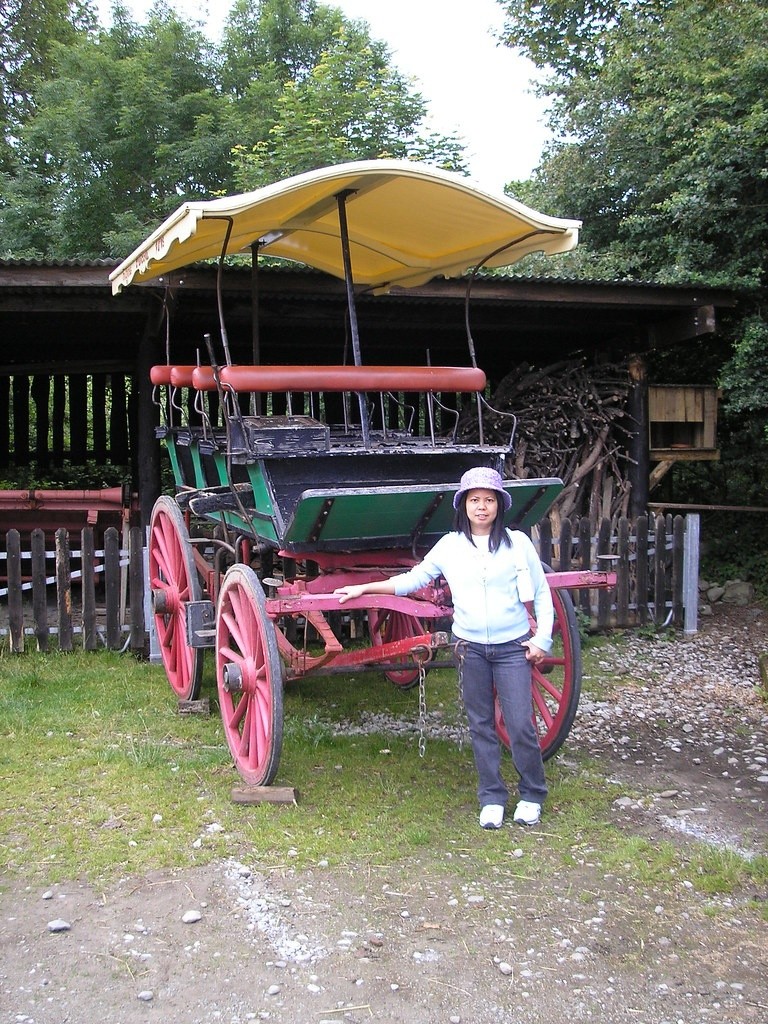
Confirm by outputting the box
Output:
[452,466,512,512]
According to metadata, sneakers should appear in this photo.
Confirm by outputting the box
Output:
[478,803,504,830]
[512,799,542,825]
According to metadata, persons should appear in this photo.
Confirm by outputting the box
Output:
[332,466,554,829]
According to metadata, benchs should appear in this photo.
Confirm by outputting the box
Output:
[154,426,564,555]
